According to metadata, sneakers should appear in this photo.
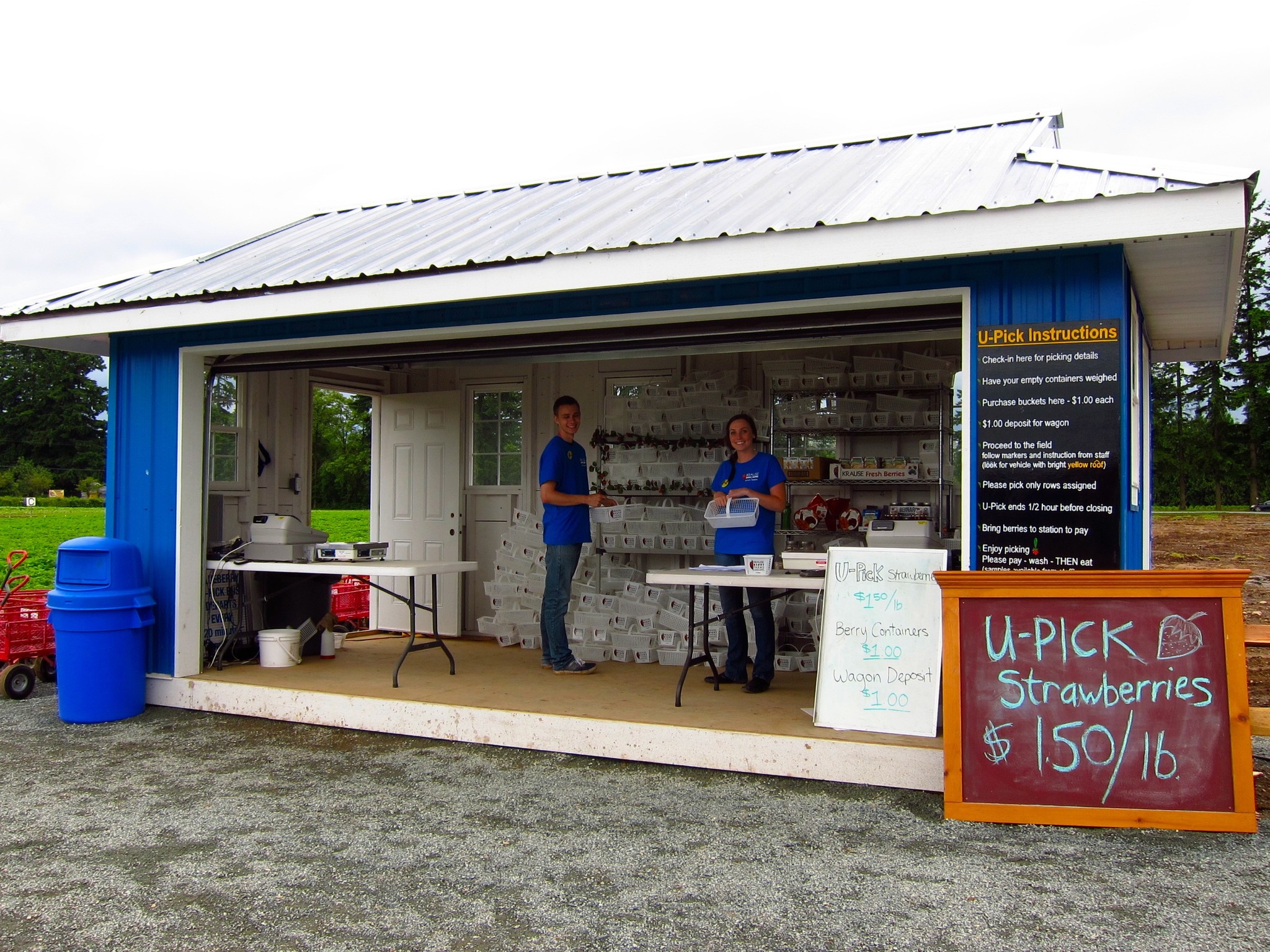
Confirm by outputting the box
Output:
[552,659,597,674]
[542,659,553,670]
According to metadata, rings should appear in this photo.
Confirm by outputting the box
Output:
[727,495,728,496]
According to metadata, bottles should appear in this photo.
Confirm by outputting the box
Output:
[320,628,336,659]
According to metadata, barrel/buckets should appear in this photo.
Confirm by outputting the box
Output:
[256,630,303,668]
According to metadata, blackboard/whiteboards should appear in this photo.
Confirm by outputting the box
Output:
[931,569,1261,834]
[811,546,949,739]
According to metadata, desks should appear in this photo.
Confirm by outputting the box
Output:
[642,570,824,708]
[206,558,478,689]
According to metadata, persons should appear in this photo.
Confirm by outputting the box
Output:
[709,413,787,694]
[538,396,617,676]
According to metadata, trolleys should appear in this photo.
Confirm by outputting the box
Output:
[1,550,57,698]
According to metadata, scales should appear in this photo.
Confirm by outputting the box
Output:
[313,542,389,563]
[782,550,827,577]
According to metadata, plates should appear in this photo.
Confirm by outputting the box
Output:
[793,507,818,530]
[840,508,860,530]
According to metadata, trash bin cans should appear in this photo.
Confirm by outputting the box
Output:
[46,536,157,724]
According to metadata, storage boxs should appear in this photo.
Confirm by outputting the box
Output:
[285,617,317,657]
[478,347,955,670]
[333,624,348,649]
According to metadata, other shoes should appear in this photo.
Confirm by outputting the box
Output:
[741,677,769,693]
[704,672,745,683]
